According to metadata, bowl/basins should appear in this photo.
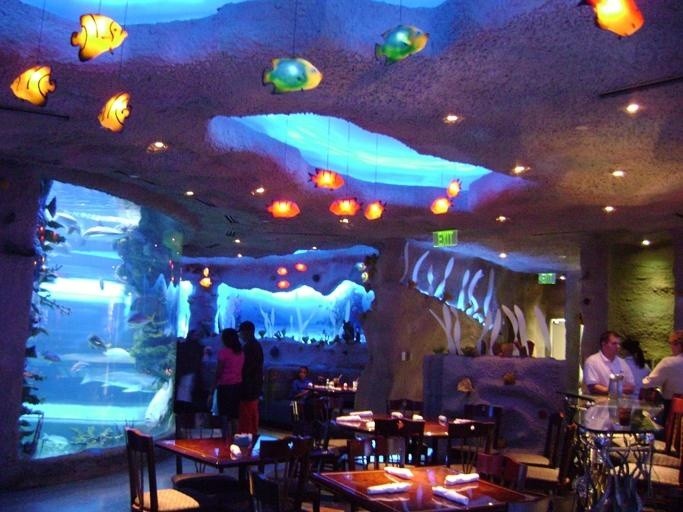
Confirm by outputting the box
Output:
[235,435,251,447]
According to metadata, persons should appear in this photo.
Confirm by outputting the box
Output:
[620,334,653,398]
[170,320,312,440]
[641,329,683,424]
[583,330,636,396]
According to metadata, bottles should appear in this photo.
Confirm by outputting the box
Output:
[326,378,329,389]
[608,368,624,396]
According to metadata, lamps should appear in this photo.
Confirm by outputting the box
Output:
[262,110,392,219]
[69,10,128,66]
[575,0,640,47]
[7,0,644,222]
[371,22,434,70]
[257,54,321,102]
[88,84,135,137]
[8,58,56,108]
[426,176,462,216]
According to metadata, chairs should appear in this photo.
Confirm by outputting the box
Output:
[123,371,681,509]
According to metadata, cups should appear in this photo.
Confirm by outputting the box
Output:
[328,382,333,389]
[343,382,347,390]
[617,404,632,425]
[352,381,357,392]
